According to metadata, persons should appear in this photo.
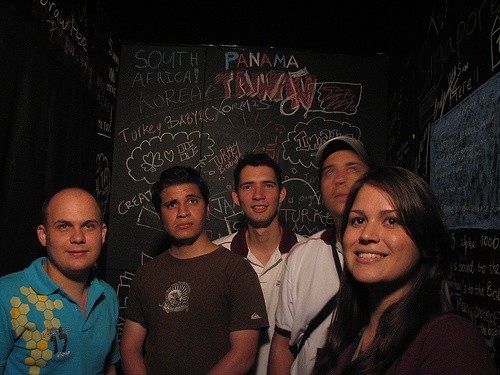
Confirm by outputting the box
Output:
[120,166,271,375]
[266,135,374,375]
[210,151,309,375]
[310,165,500,375]
[0,186,123,375]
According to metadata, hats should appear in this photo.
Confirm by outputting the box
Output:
[314,135,369,168]
